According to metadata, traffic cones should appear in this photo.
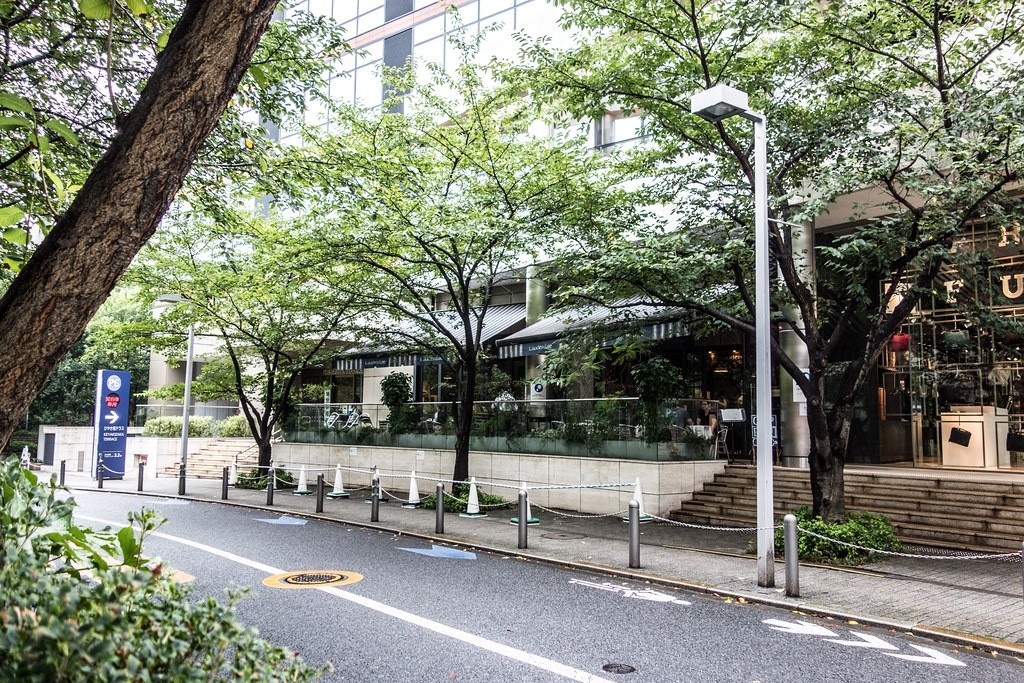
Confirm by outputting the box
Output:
[400,470,426,509]
[511,482,540,525]
[228,460,238,484]
[365,468,389,504]
[291,465,313,496]
[459,477,488,518]
[623,476,653,524]
[265,467,279,490]
[327,462,350,499]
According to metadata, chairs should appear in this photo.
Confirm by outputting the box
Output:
[325,412,388,429]
[715,426,729,460]
[670,424,687,441]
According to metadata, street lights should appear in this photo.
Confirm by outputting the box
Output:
[160,294,195,467]
[690,83,776,588]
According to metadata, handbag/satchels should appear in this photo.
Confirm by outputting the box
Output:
[1002,330,1024,348]
[889,332,913,352]
[945,329,970,347]
[1006,432,1024,453]
[948,427,971,447]
[883,362,1024,414]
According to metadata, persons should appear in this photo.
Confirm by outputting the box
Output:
[695,401,722,444]
[431,404,444,432]
[668,401,692,442]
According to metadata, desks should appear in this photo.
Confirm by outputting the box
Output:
[689,425,712,439]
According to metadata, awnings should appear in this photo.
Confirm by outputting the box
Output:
[336,301,525,372]
[495,279,752,359]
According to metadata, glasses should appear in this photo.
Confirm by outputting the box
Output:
[701,403,708,406]
